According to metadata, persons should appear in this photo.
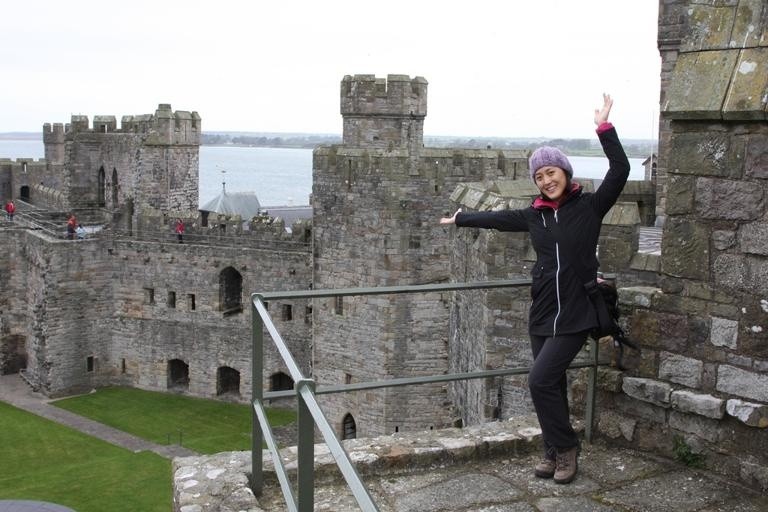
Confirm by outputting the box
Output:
[9,202,14,220]
[176,220,184,244]
[6,202,10,219]
[439,92,631,483]
[66,214,85,239]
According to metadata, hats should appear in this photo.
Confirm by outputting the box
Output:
[529,147,573,186]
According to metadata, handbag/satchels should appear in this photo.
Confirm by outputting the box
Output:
[585,277,621,340]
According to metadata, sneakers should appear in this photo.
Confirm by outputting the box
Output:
[534,442,580,483]
